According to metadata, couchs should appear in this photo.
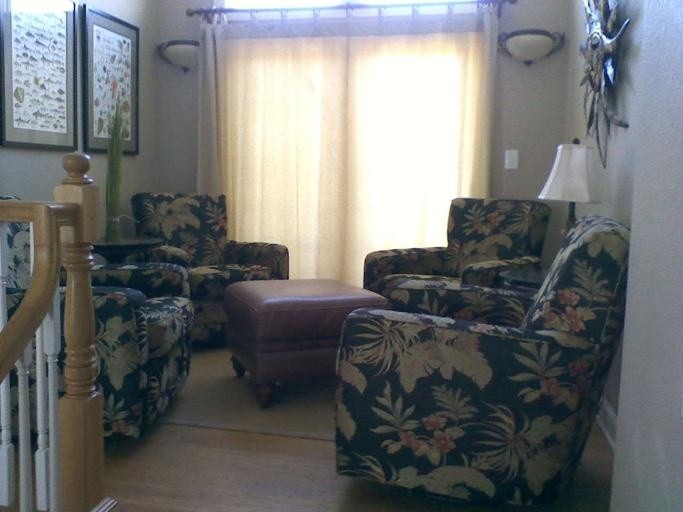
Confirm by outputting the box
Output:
[222,275,393,410]
[131,190,288,354]
[363,196,554,287]
[0,195,194,453]
[334,212,630,512]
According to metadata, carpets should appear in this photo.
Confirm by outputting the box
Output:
[157,345,335,445]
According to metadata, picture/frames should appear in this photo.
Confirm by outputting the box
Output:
[81,3,141,154]
[0,0,79,152]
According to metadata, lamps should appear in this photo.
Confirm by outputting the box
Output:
[155,40,201,74]
[537,138,606,225]
[496,28,567,66]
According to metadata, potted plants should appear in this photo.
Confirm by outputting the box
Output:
[105,93,125,240]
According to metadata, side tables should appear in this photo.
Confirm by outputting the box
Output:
[499,267,548,291]
[87,236,165,264]
